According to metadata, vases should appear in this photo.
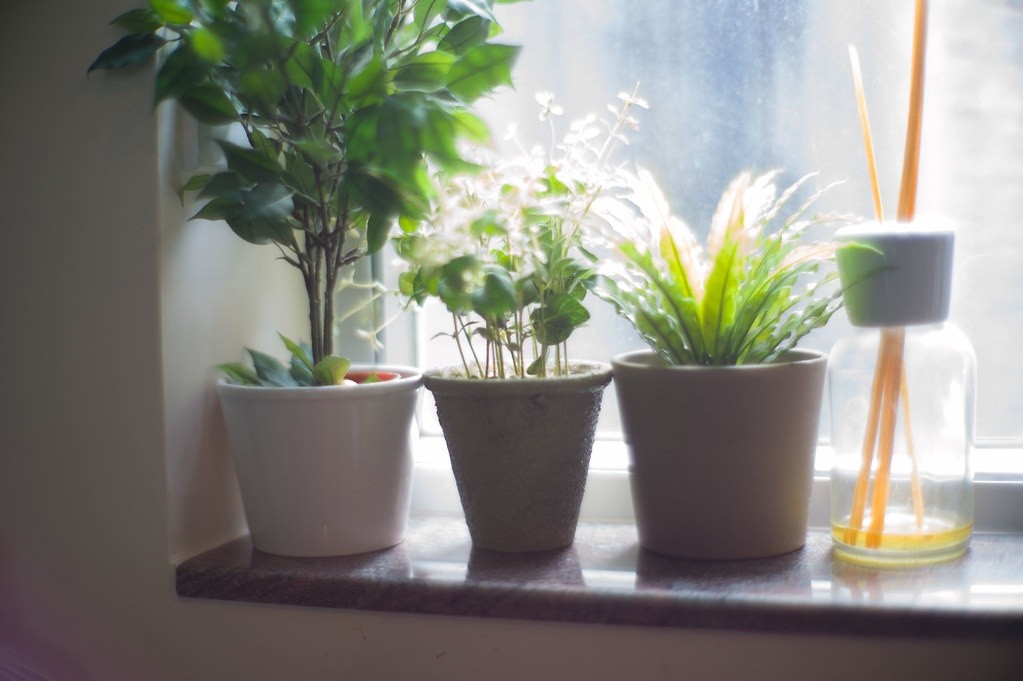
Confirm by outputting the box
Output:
[820,229,975,568]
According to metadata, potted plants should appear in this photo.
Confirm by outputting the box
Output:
[580,156,908,568]
[80,0,521,560]
[387,141,641,556]
